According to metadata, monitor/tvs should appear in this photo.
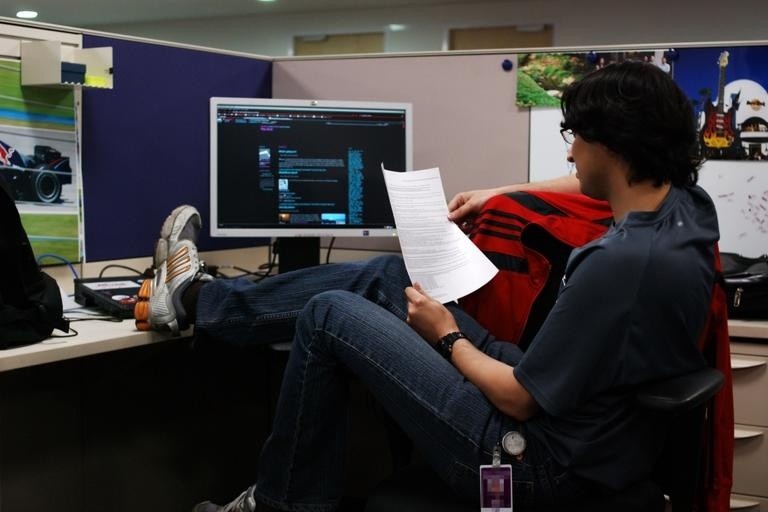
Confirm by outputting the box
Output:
[210,97,413,275]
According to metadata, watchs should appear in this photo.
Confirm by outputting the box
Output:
[435,332,471,360]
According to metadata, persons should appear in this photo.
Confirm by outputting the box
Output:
[133,60,720,512]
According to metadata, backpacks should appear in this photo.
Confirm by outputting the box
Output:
[1,184,69,349]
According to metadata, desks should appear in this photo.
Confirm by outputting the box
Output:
[0,293,768,509]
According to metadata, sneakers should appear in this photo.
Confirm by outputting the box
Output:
[134,204,214,337]
[191,485,257,512]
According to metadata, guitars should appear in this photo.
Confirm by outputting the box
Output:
[699,51,741,148]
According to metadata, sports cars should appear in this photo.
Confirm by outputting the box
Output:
[0,140,72,203]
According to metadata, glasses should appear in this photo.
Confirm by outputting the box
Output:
[559,123,575,143]
